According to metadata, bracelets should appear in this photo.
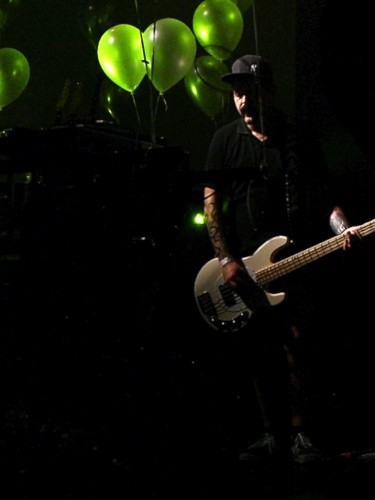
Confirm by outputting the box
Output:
[220,255,233,268]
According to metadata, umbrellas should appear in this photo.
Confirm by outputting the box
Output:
[96,0,244,120]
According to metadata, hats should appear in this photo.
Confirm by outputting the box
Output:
[220,55,272,87]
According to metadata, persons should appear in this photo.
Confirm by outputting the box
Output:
[203,54,364,465]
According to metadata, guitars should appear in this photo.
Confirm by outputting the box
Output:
[194,219,375,335]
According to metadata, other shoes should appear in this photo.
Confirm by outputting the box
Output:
[248,431,284,456]
[289,433,323,463]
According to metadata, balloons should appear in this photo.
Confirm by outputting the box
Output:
[0,47,31,111]
